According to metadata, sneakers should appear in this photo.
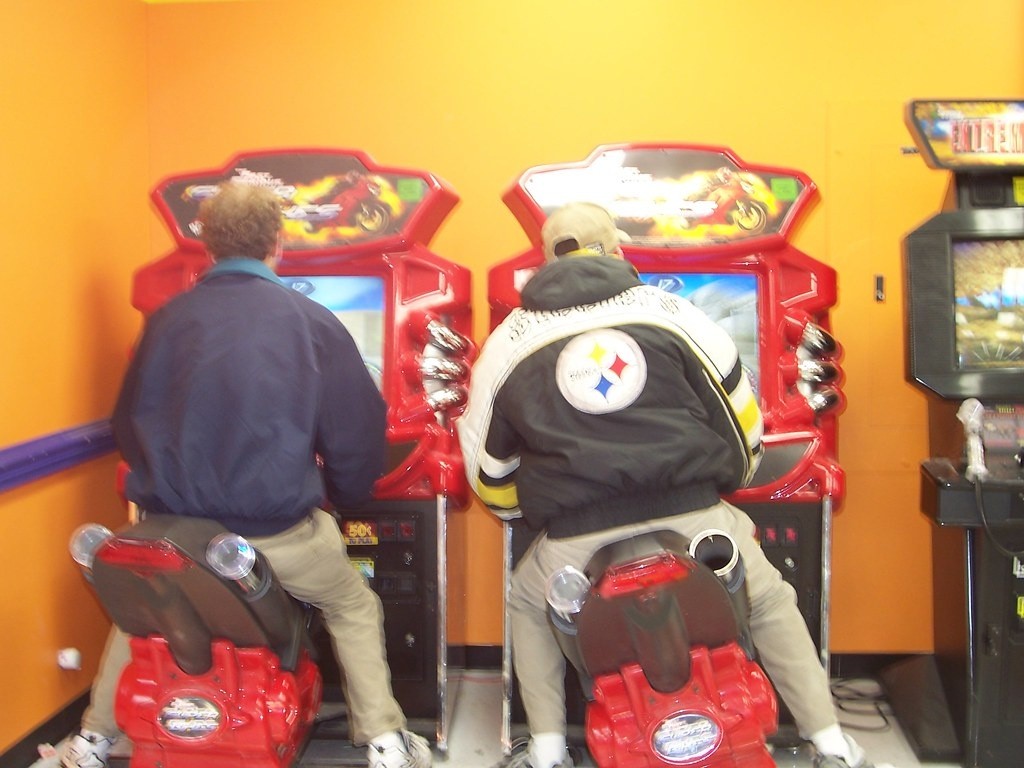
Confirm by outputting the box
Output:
[366,727,431,768]
[506,749,574,768]
[59,725,119,768]
[807,732,874,768]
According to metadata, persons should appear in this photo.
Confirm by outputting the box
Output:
[456,202,867,768]
[57,178,433,768]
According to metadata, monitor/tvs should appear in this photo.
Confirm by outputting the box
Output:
[953,238,1024,369]
[274,276,383,392]
[635,270,761,406]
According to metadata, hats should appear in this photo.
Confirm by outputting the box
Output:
[542,203,632,263]
